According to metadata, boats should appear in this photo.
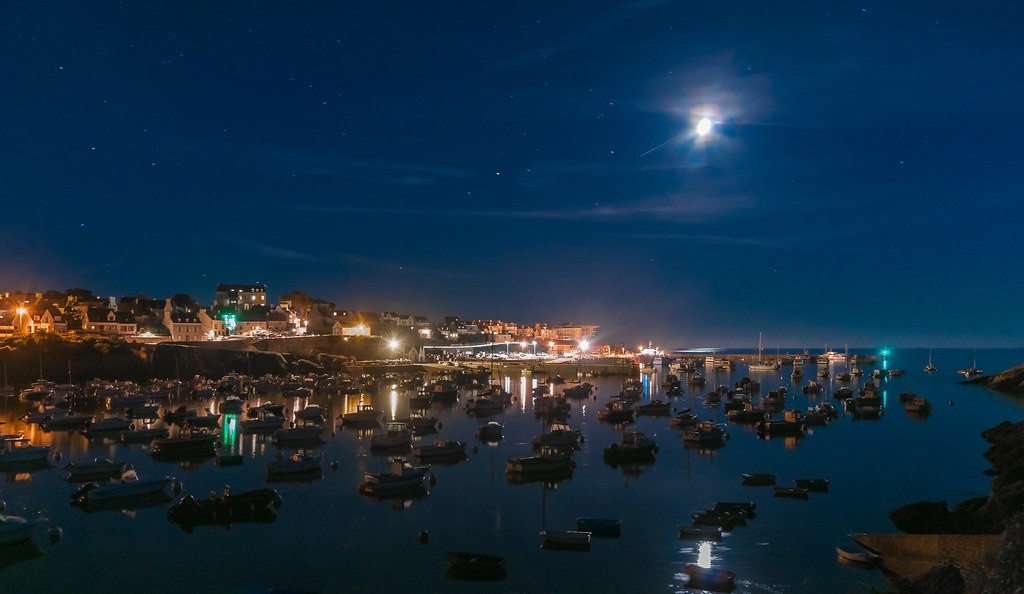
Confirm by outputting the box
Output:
[835,546,869,562]
[446,549,509,572]
[538,528,592,546]
[685,564,735,585]
[0,345,988,540]
[361,454,432,486]
[507,439,575,474]
[60,456,133,479]
[577,518,623,535]
[180,486,281,518]
[77,471,171,501]
[0,499,49,546]
